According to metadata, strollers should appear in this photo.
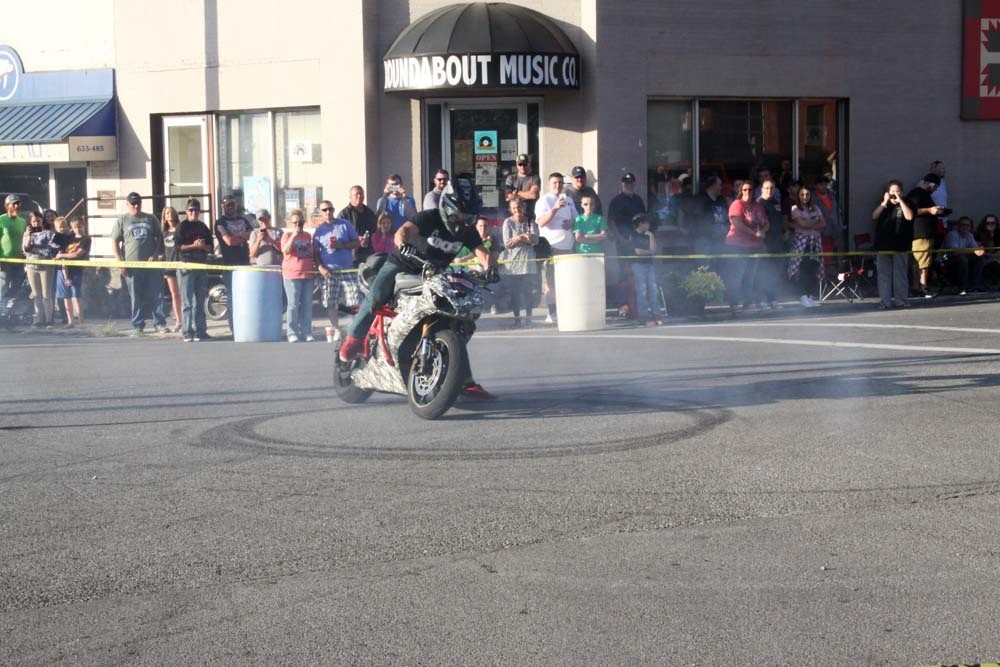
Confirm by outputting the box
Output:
[0,272,37,327]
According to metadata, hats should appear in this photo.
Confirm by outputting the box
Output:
[127,191,141,205]
[185,196,268,219]
[4,194,22,204]
[923,173,940,186]
[516,154,637,183]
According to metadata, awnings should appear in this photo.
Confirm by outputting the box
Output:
[0,97,116,163]
[381,3,579,90]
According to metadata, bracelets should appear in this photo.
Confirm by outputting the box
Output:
[880,203,887,208]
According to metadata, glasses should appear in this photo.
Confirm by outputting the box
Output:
[291,220,306,224]
[742,188,753,191]
[986,222,996,225]
[435,177,447,181]
[960,223,971,227]
[320,207,334,212]
[584,202,595,206]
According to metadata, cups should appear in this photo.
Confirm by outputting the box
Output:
[559,193,567,207]
[329,236,337,249]
[325,326,336,342]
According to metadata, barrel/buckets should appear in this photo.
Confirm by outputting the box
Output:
[553,252,606,332]
[230,265,283,342]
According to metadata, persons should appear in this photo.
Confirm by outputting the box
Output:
[340,176,495,402]
[564,167,602,215]
[650,158,691,216]
[534,173,579,322]
[871,179,917,309]
[823,168,835,187]
[917,160,948,230]
[788,184,826,307]
[0,195,91,329]
[111,168,500,344]
[609,173,647,301]
[573,194,608,253]
[628,213,665,326]
[906,173,943,298]
[505,154,542,216]
[813,177,844,273]
[677,157,790,309]
[502,200,539,328]
[943,215,1000,297]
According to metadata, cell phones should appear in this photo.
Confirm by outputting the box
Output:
[260,229,266,232]
[298,220,303,227]
[30,227,42,232]
[889,194,896,199]
[559,194,566,207]
[388,185,401,191]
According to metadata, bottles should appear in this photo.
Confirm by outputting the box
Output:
[363,230,370,248]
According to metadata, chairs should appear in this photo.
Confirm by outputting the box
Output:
[819,234,880,304]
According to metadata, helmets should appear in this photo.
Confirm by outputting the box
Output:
[438,177,483,239]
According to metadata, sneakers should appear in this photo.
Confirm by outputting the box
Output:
[339,337,364,362]
[460,384,498,402]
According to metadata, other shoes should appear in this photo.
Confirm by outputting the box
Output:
[512,320,533,330]
[31,323,54,330]
[131,323,205,341]
[288,326,341,344]
[728,294,821,313]
[878,286,932,310]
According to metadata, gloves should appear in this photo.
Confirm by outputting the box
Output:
[482,267,500,284]
[399,242,418,259]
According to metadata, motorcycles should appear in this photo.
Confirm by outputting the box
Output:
[333,250,501,420]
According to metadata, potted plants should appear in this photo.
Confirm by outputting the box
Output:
[683,270,724,315]
